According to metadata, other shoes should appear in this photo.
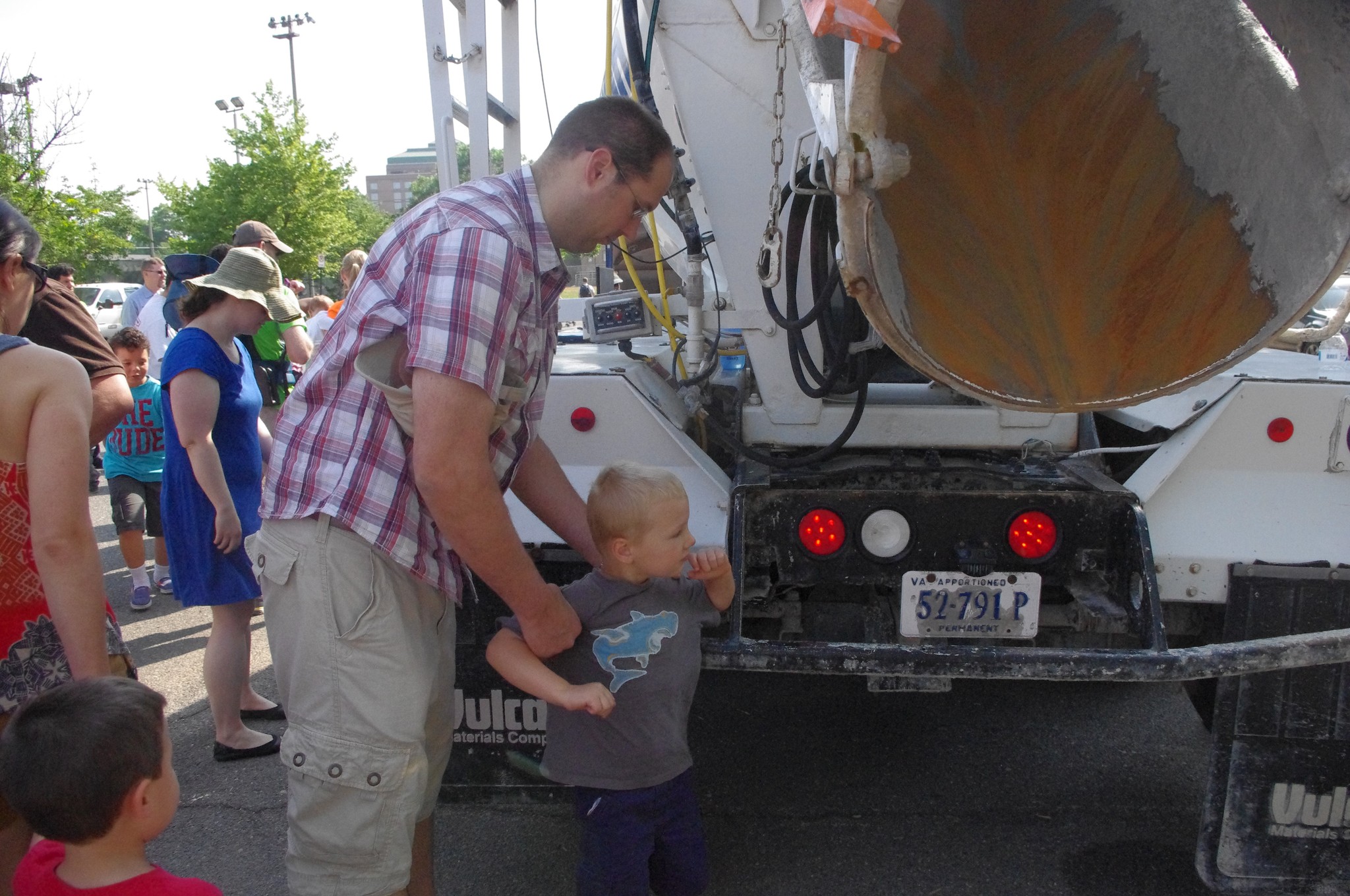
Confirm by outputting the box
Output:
[214,731,282,759]
[240,702,286,720]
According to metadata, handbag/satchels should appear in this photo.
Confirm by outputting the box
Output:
[253,360,281,407]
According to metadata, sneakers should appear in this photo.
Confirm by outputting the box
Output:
[153,577,174,594]
[130,581,152,609]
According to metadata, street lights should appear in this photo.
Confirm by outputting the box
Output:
[137,176,160,258]
[216,95,248,166]
[1,73,42,191]
[269,13,316,134]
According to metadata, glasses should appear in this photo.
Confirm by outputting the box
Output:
[145,270,167,275]
[585,146,648,221]
[22,259,48,295]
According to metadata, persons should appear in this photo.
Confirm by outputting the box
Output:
[0,675,224,896]
[484,459,735,896]
[240,94,680,895]
[17,276,135,453]
[100,325,177,613]
[1,199,141,894]
[122,218,374,497]
[47,262,105,491]
[160,248,287,762]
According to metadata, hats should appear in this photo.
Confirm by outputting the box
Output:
[233,220,294,256]
[354,332,528,440]
[181,247,306,324]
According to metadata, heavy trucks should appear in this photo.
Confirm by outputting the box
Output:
[417,1,1349,894]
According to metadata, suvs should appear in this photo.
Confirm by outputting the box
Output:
[72,282,145,339]
